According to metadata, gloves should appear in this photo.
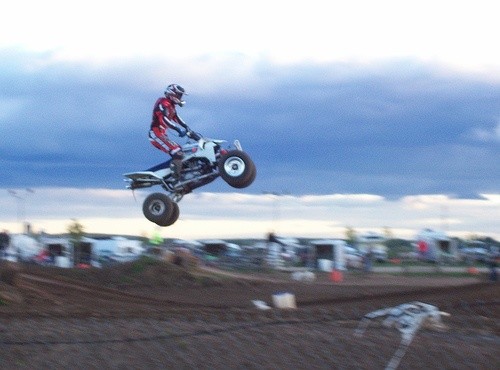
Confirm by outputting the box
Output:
[179,129,185,137]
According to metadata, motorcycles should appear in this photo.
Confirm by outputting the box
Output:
[122,130,257,227]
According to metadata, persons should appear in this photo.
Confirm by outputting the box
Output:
[148,84,200,184]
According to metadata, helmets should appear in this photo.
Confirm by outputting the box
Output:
[164,84,188,108]
